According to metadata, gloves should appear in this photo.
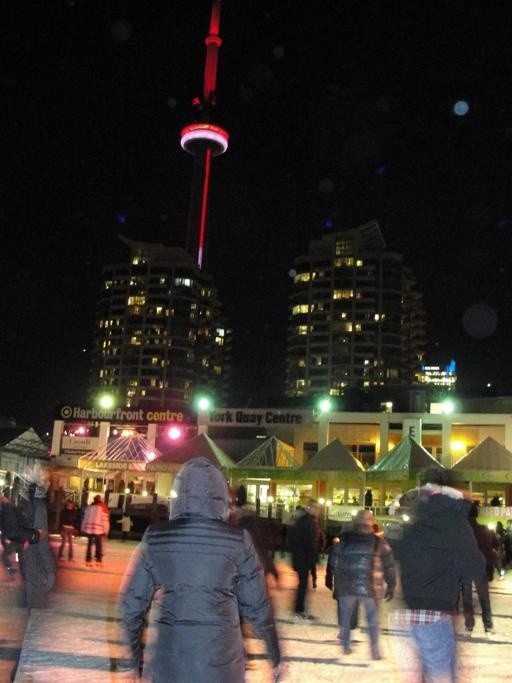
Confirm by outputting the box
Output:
[326,580,333,591]
[384,591,394,602]
[29,531,40,544]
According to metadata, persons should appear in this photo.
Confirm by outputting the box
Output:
[0,464,512,683]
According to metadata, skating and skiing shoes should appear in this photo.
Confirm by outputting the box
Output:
[58,559,105,569]
[465,624,496,638]
[291,611,316,625]
